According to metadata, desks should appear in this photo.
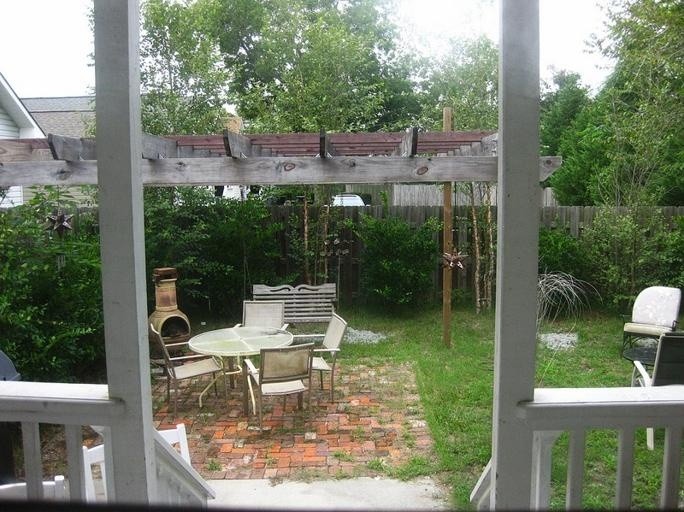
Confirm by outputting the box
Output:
[623,348,657,370]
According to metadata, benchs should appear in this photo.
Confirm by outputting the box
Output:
[253,283,337,323]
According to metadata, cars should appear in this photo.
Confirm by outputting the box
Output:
[324,194,371,207]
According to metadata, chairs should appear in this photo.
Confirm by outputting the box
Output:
[82,423,192,505]
[150,301,347,433]
[623,285,682,353]
[631,332,684,451]
